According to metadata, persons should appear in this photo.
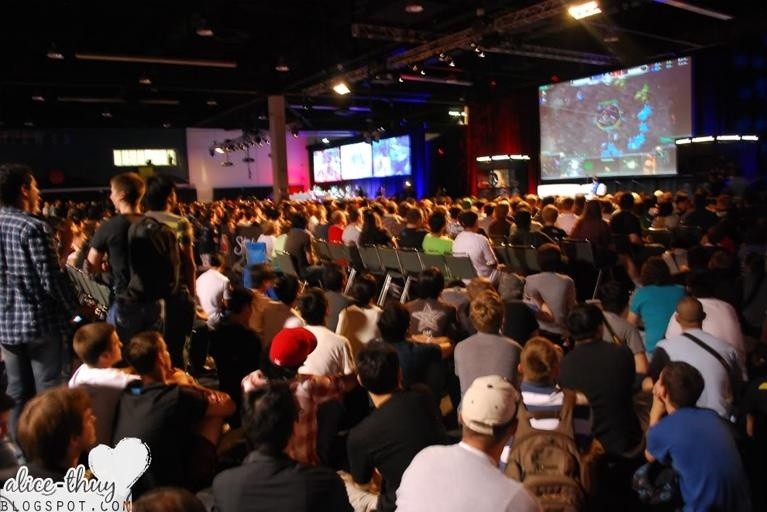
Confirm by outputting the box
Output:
[0,164,766,511]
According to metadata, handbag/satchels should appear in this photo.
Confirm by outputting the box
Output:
[632,461,676,505]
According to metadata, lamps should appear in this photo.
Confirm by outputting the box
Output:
[448,104,470,125]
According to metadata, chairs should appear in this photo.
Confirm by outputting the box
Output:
[62,264,112,310]
[230,221,727,311]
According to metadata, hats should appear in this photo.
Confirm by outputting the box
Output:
[460,374,521,434]
[269,327,317,368]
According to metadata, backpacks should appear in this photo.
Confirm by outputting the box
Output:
[505,388,589,512]
[122,213,180,305]
